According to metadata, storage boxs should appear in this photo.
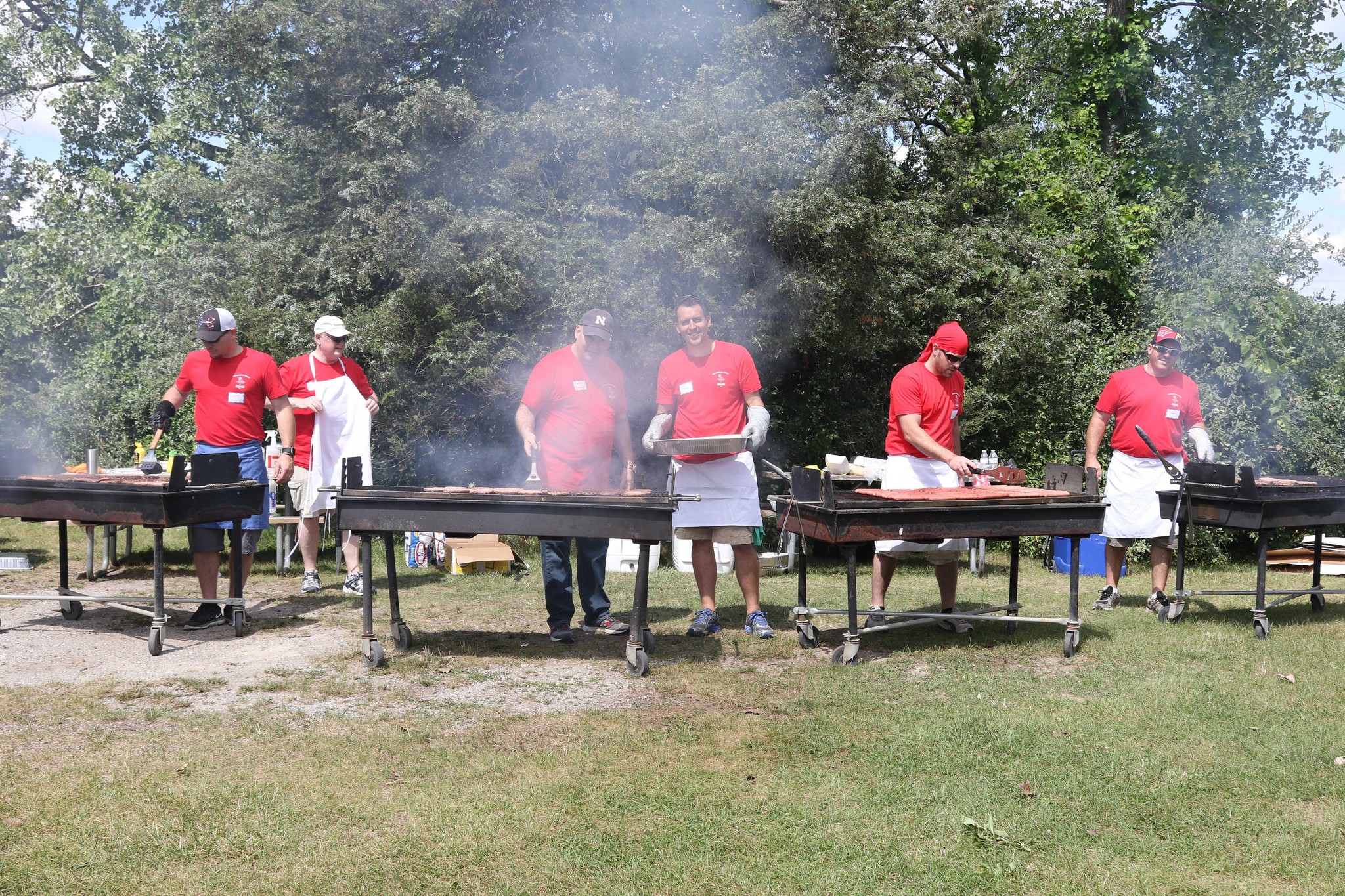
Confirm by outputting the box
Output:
[420,533,530,575]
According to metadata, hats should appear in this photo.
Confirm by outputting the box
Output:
[314,315,355,338]
[1152,325,1183,348]
[191,307,236,342]
[580,309,615,343]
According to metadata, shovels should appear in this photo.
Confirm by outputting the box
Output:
[135,420,166,470]
[967,465,1025,483]
[1135,425,1183,480]
[526,443,542,481]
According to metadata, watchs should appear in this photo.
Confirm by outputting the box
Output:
[621,464,637,474]
[282,447,295,456]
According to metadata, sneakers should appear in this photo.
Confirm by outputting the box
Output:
[865,605,888,632]
[342,571,377,596]
[301,570,322,593]
[223,605,252,623]
[745,610,774,639]
[937,606,975,634]
[1145,590,1170,617]
[687,608,720,636]
[1091,585,1122,610]
[582,616,631,634]
[182,603,225,630]
[549,622,575,643]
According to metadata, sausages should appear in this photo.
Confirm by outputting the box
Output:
[855,485,1070,501]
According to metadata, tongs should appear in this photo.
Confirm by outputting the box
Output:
[776,494,807,557]
[1168,473,1195,545]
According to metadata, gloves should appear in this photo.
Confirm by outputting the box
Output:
[149,400,176,440]
[740,404,770,453]
[1186,427,1215,464]
[642,412,673,456]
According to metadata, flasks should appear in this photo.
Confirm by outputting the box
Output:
[85,449,98,474]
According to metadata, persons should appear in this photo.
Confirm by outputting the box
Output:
[1085,326,1215,617]
[514,309,637,642]
[865,321,977,634]
[264,315,380,596]
[641,294,775,639]
[151,307,296,631]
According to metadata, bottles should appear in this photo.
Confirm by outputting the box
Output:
[989,450,998,478]
[980,450,989,477]
[134,443,145,468]
[166,456,174,473]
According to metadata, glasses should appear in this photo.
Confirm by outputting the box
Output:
[200,329,231,344]
[1152,344,1182,356]
[674,295,702,305]
[941,349,967,363]
[320,334,349,343]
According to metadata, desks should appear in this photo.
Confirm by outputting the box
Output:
[761,471,985,578]
[43,516,325,580]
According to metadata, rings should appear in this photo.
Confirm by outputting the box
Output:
[631,481,634,483]
[288,475,291,478]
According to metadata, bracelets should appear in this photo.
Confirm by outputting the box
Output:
[947,454,956,465]
[281,453,293,457]
